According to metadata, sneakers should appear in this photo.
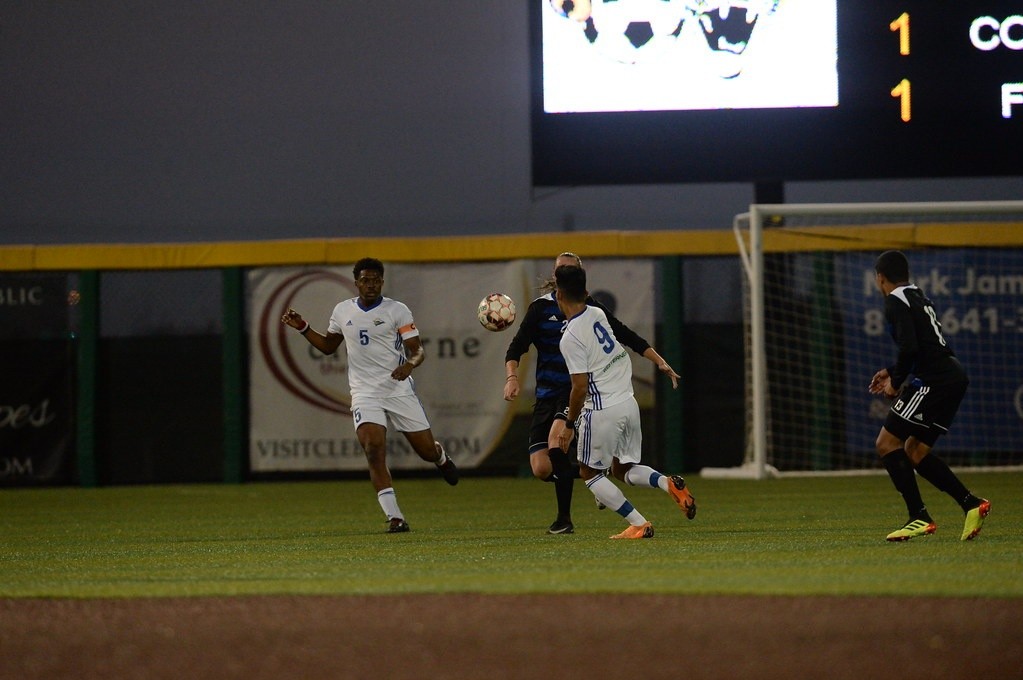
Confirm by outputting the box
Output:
[667,475,697,520]
[609,521,654,539]
[384,518,409,533]
[886,516,938,541]
[435,440,459,486]
[595,466,611,510]
[960,498,991,540]
[548,521,574,534]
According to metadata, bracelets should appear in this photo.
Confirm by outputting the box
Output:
[506,374,517,379]
[566,418,575,429]
[297,321,308,333]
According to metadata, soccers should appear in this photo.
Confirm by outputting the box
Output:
[476,292,517,333]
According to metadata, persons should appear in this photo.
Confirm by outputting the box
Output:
[502,251,681,535]
[553,264,697,539]
[868,248,992,540]
[280,258,460,533]
